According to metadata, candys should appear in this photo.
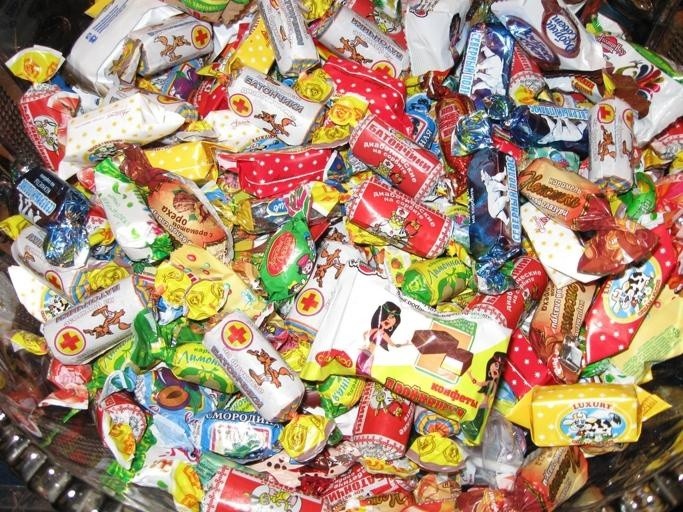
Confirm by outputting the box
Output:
[1,1,683,511]
